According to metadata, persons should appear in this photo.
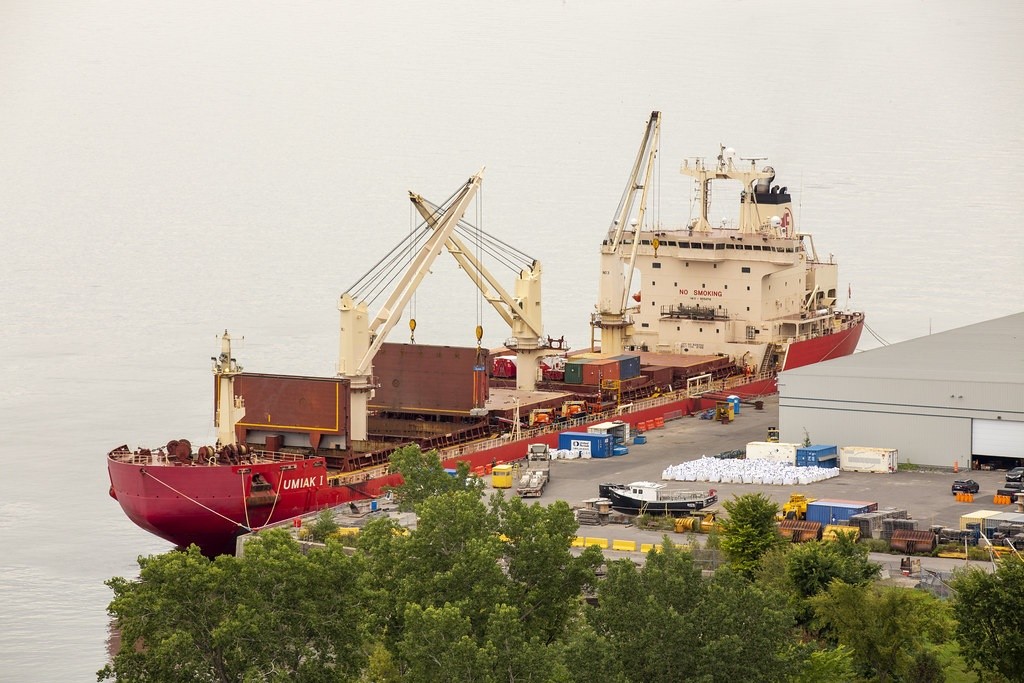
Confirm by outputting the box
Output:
[157,449,165,462]
[743,362,758,377]
[901,557,910,570]
[974,459,979,470]
[492,462,497,467]
[800,305,807,330]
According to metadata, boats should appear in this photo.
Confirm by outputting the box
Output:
[105,113,866,548]
[608,481,719,518]
[632,290,641,303]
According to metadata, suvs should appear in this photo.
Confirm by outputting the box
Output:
[952,478,980,496]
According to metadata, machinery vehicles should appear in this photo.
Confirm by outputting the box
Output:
[773,493,817,522]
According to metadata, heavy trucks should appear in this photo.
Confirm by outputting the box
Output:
[517,442,552,498]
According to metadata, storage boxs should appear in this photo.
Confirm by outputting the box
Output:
[746,441,898,474]
[960,510,1024,538]
[806,498,878,527]
[493,355,641,385]
[559,421,630,458]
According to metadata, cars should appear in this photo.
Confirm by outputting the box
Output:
[1005,467,1024,482]
